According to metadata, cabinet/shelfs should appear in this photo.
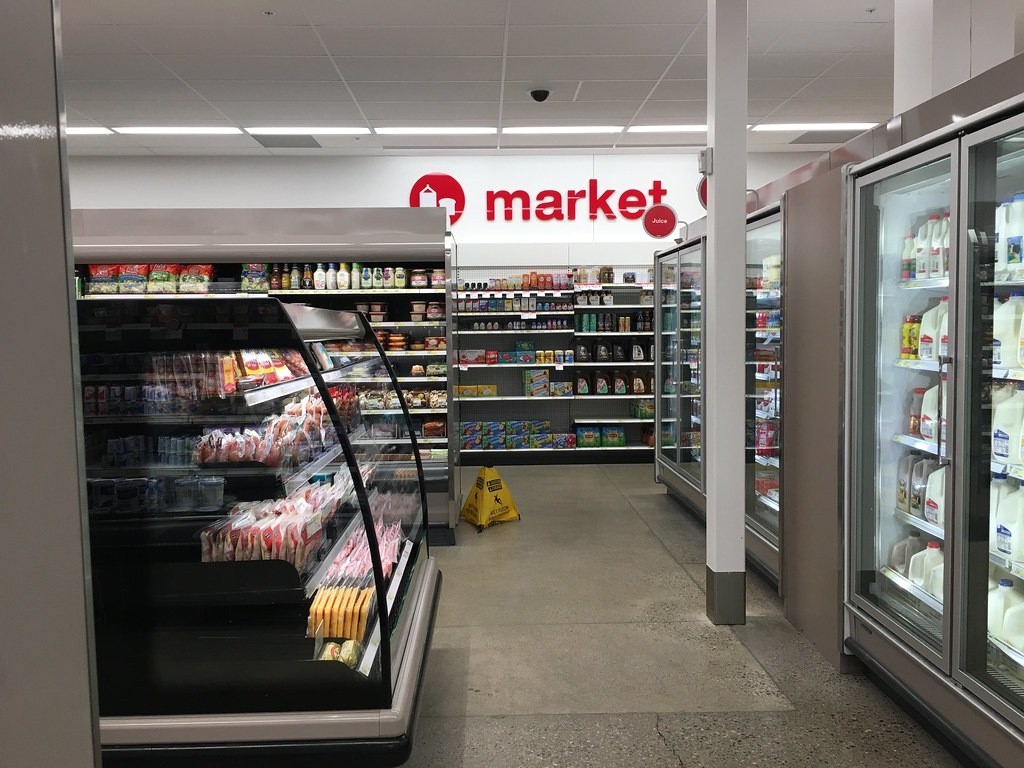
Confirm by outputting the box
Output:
[76,297,444,768]
[237,289,449,494]
[452,264,655,467]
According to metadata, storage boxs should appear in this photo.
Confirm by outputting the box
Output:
[498,351,517,364]
[516,351,536,364]
[458,369,655,450]
[485,350,498,364]
[459,349,485,364]
[457,271,575,291]
[515,340,536,351]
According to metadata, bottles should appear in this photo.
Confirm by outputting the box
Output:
[573,266,614,282]
[575,336,655,362]
[902,289,1023,370]
[427,302,446,321]
[637,311,655,331]
[575,289,654,305]
[630,399,656,418]
[536,301,573,311]
[640,424,675,446]
[891,532,1024,652]
[574,369,655,394]
[576,426,626,447]
[899,193,1024,279]
[896,451,1024,562]
[270,263,447,289]
[755,418,777,456]
[473,320,568,331]
[574,312,631,333]
[910,376,1023,465]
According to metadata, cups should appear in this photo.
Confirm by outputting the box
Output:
[174,477,225,508]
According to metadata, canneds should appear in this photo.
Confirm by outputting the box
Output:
[411,270,428,288]
[573,313,630,332]
[513,320,568,330]
[536,350,575,363]
[431,269,446,288]
[537,302,574,310]
[488,272,567,290]
[427,302,445,321]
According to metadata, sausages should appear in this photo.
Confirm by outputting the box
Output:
[196,393,360,469]
